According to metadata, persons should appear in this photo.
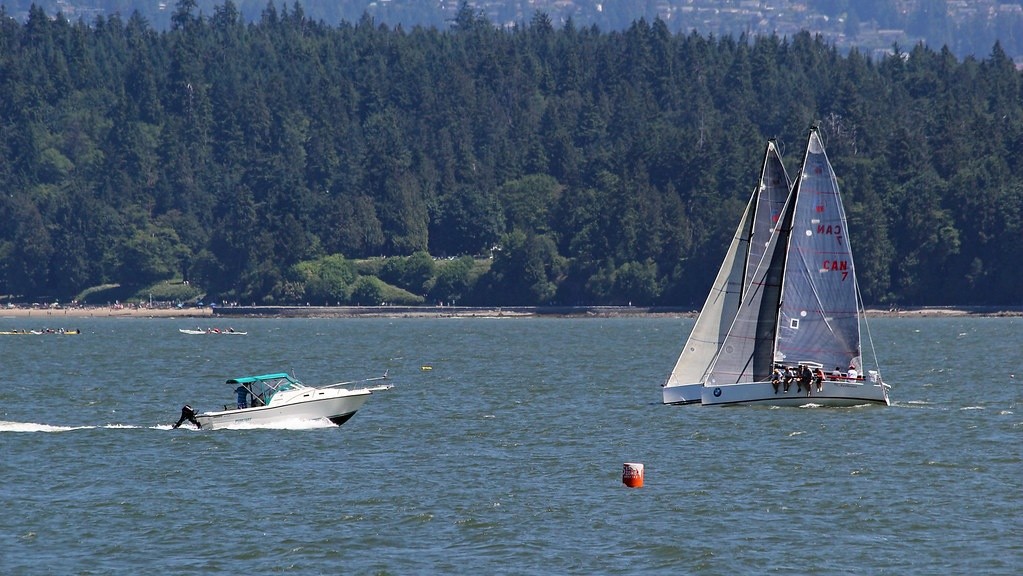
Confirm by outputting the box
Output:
[0,281,457,319]
[234,383,252,409]
[830,367,841,381]
[772,369,782,394]
[845,364,858,383]
[224,327,234,332]
[205,327,211,335]
[781,367,794,393]
[815,368,826,392]
[379,250,489,262]
[628,301,632,307]
[195,325,202,331]
[213,328,221,334]
[11,327,69,335]
[796,364,814,397]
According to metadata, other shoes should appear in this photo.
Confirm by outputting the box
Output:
[784,389,788,392]
[817,388,820,393]
[798,388,801,393]
[775,390,778,394]
[820,387,822,392]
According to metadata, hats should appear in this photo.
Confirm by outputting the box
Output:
[849,365,855,369]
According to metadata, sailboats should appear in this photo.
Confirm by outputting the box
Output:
[661,119,895,407]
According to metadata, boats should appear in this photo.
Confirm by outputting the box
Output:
[179,327,248,335]
[0,329,81,335]
[168,367,395,432]
